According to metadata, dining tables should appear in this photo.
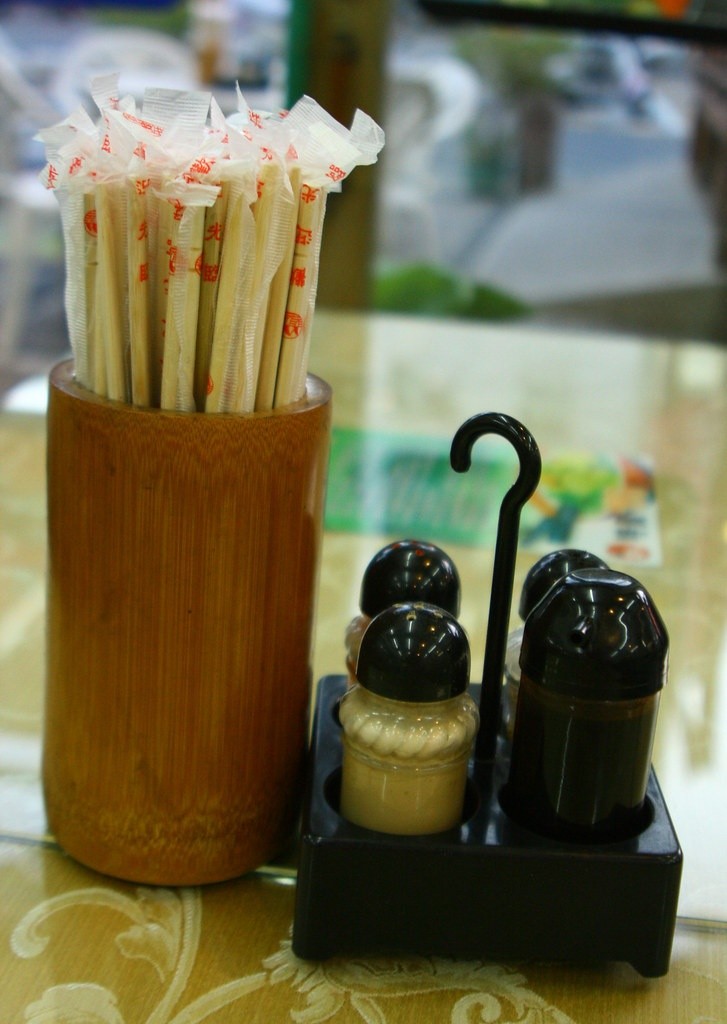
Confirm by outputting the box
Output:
[2,305,727,1024]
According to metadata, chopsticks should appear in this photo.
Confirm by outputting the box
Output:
[83,162,327,412]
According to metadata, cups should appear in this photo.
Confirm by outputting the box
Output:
[42,358,332,887]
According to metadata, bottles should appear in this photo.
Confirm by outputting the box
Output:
[338,603,480,834]
[345,540,459,688]
[505,549,611,737]
[509,567,669,827]
